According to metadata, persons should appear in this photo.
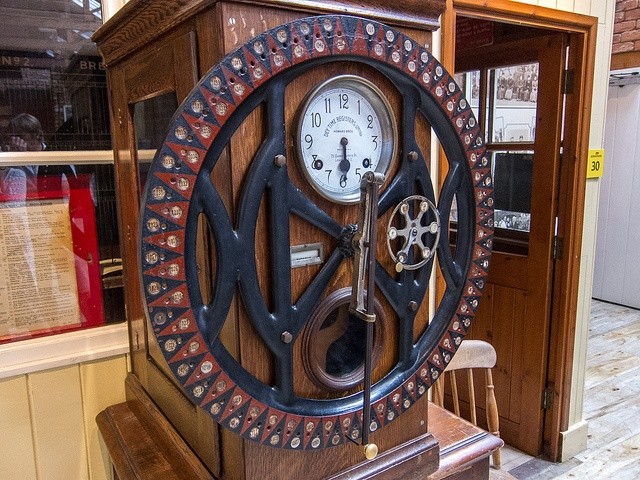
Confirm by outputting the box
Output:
[507,137,517,153]
[0,165,42,345]
[0,112,77,192]
[494,128,502,143]
[472,64,538,102]
[494,214,529,231]
[517,136,527,153]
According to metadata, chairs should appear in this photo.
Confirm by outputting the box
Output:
[433,340,519,480]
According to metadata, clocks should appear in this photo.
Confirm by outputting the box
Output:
[296,74,399,206]
